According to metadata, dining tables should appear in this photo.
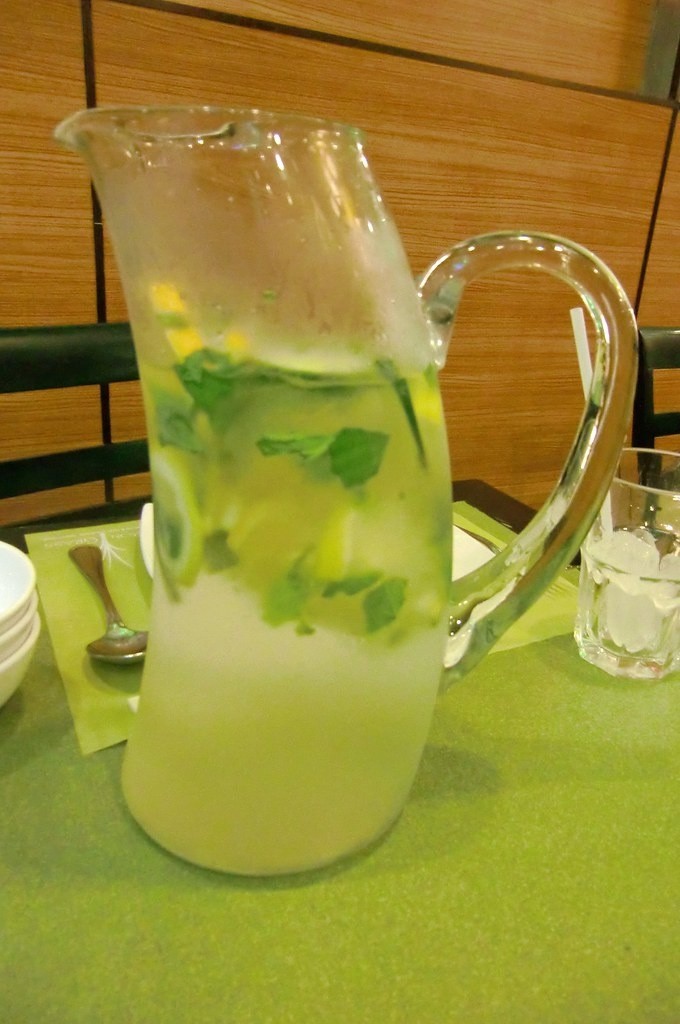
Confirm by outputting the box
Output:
[0,478,680,1023]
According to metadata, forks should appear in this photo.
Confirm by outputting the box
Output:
[453,523,568,599]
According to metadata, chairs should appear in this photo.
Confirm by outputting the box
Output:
[0,322,154,523]
[631,327,680,537]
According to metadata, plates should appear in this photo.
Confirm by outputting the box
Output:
[141,501,521,667]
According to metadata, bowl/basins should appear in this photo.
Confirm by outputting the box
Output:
[0,541,42,712]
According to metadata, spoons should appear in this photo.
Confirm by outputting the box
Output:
[68,545,150,665]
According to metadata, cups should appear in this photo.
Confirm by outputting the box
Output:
[52,105,638,877]
[573,449,680,680]
